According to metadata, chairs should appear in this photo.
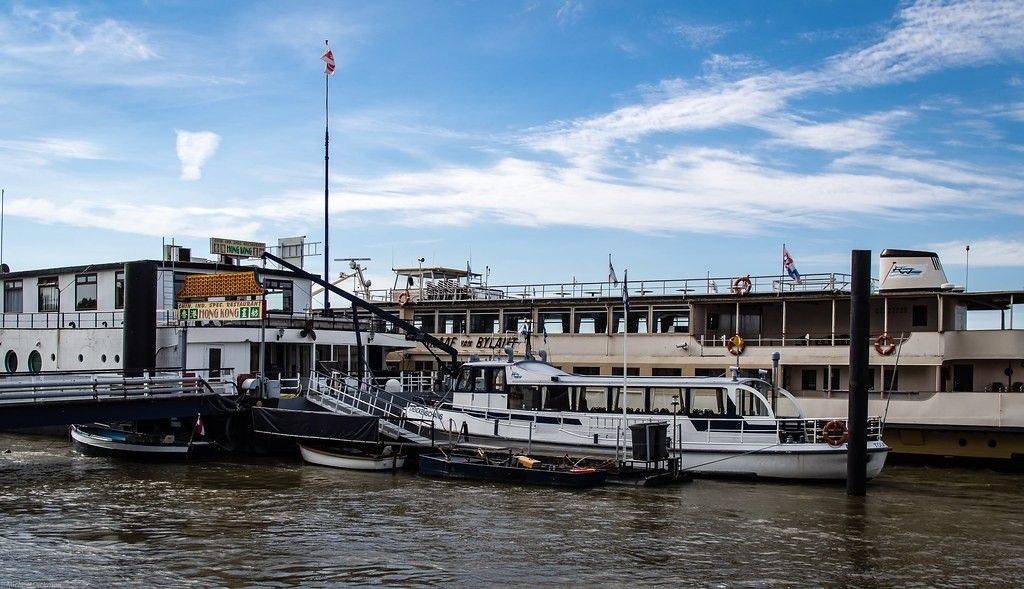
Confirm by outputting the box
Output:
[794,334,850,346]
[985,381,1024,392]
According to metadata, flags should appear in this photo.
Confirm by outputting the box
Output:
[608,263,618,288]
[623,276,630,312]
[195,417,205,435]
[709,274,717,293]
[321,44,336,76]
[784,247,800,282]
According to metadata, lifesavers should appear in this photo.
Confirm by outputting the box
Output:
[398,291,409,306]
[874,334,896,355]
[727,336,745,355]
[823,420,848,446]
[734,277,751,295]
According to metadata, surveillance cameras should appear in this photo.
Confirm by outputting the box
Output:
[676,343,687,348]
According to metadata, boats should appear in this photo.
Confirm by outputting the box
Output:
[381,331,891,483]
[68,375,222,463]
[417,448,608,490]
[0,190,463,392]
[297,438,408,470]
[384,241,1024,467]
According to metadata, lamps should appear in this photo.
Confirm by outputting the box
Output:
[368,330,375,343]
[277,327,285,340]
[36,341,41,348]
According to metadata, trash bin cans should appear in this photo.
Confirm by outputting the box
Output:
[266,380,281,398]
[628,422,670,460]
